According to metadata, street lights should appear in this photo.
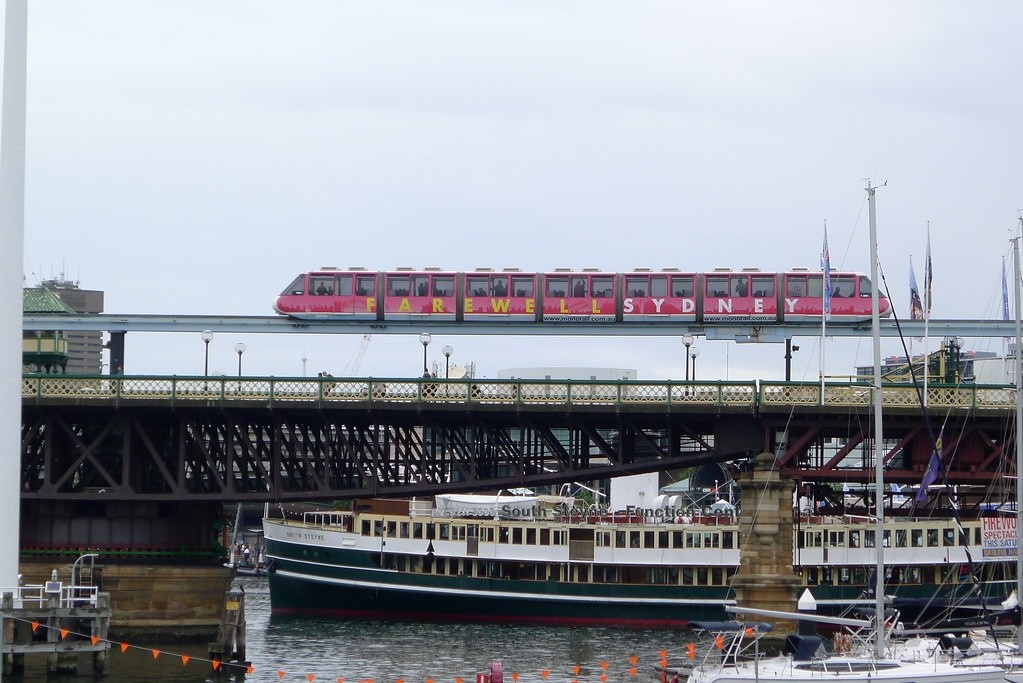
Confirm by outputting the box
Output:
[681,333,694,401]
[689,346,700,396]
[953,337,965,403]
[441,345,453,403]
[419,333,431,376]
[201,329,213,396]
[234,342,246,395]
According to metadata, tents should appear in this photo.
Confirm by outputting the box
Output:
[660,476,741,494]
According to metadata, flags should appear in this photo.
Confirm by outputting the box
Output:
[910,246,933,320]
[916,428,944,503]
[1002,269,1010,339]
[824,237,831,319]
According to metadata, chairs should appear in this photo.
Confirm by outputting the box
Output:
[365,288,795,297]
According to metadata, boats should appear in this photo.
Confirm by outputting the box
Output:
[261,479,1022,631]
[223,562,269,577]
[435,493,540,521]
[653,180,1023,683]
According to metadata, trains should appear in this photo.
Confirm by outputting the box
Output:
[273,267,894,331]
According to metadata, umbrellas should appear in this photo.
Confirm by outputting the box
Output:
[701,499,740,515]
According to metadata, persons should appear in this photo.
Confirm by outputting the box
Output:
[423,367,437,397]
[234,542,266,579]
[322,371,335,397]
[316,278,851,297]
